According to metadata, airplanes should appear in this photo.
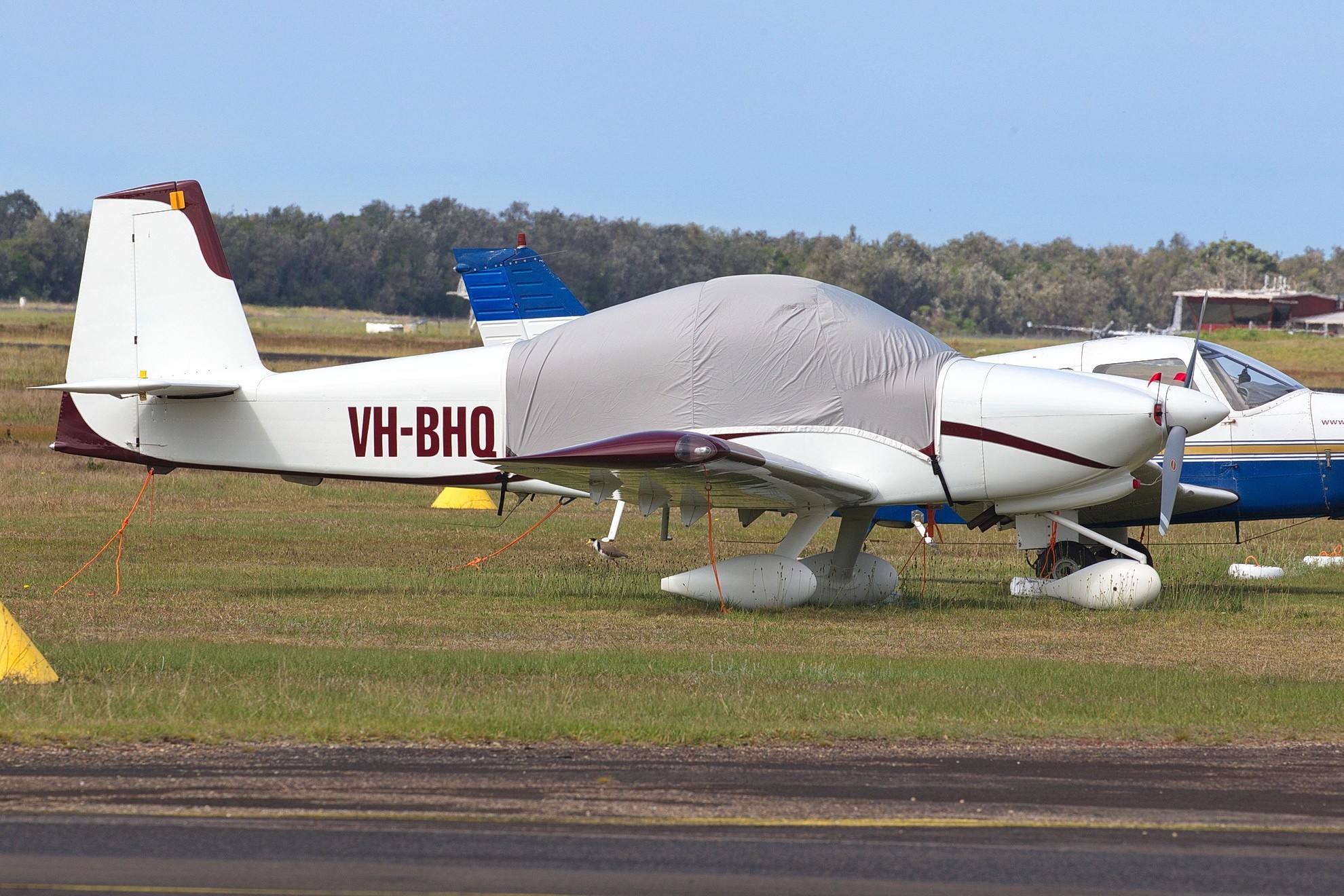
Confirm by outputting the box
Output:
[28,179,1242,609]
[736,334,1343,577]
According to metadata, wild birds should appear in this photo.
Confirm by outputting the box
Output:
[585,538,628,568]
